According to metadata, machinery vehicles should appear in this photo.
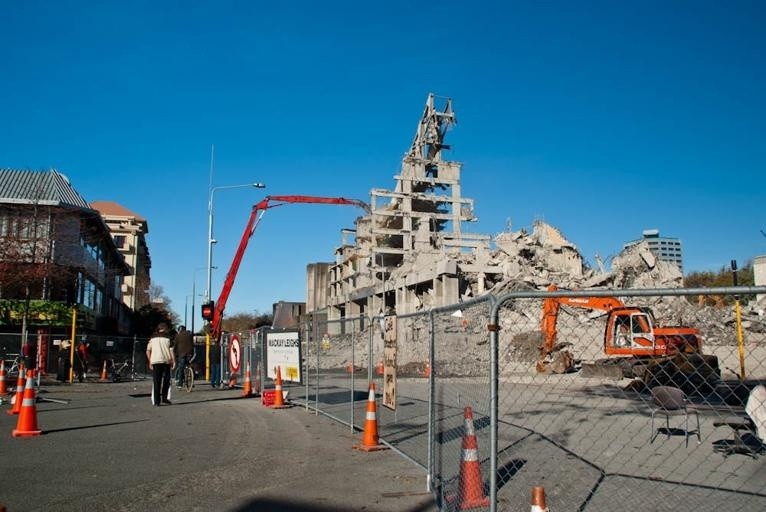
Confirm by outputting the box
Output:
[699,293,730,308]
[195,196,372,374]
[536,284,721,390]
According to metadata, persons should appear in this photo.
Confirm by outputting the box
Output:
[21,338,35,368]
[616,323,633,349]
[146,322,195,405]
[75,332,91,380]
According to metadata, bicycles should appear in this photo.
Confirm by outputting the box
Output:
[173,351,194,394]
[109,359,137,383]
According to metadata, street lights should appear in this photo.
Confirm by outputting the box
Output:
[205,182,265,381]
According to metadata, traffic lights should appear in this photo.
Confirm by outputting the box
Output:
[200,302,213,319]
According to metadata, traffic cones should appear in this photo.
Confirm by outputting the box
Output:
[354,381,389,452]
[242,361,262,396]
[99,360,108,382]
[0,358,43,437]
[425,350,430,378]
[273,366,286,409]
[530,484,546,512]
[377,357,384,376]
[229,374,237,389]
[454,406,490,509]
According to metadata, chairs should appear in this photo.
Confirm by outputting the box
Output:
[714,385,765,459]
[647,385,702,446]
